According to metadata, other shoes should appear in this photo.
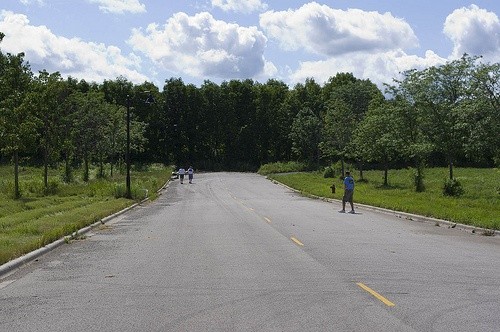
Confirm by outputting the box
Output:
[338,210,345,213]
[348,210,355,214]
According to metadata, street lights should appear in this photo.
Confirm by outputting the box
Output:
[125,90,154,199]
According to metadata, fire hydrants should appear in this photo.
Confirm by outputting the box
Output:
[330,184,335,194]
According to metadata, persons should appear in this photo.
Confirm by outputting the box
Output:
[188,166,193,184]
[338,171,355,214]
[179,166,186,184]
